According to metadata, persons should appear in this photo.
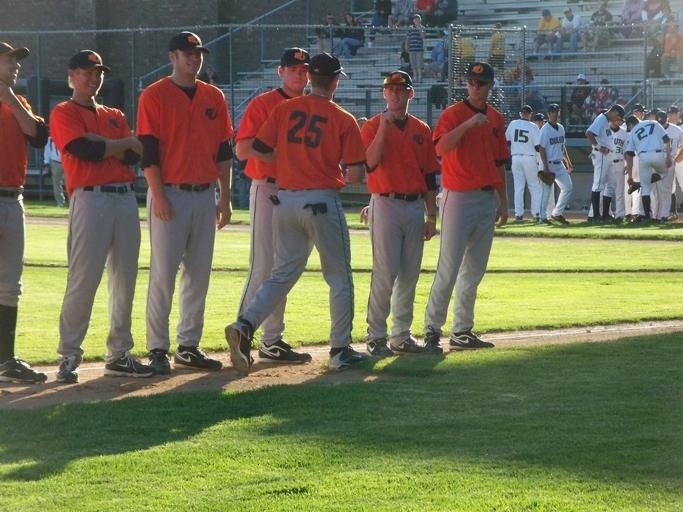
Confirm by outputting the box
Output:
[505,103,683,225]
[43,133,70,209]
[235,46,313,365]
[0,41,47,386]
[224,51,370,377]
[359,70,440,358]
[200,63,219,86]
[48,49,155,383]
[318,0,682,128]
[135,30,236,375]
[425,61,511,354]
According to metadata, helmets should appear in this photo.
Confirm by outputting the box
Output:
[70,50,112,73]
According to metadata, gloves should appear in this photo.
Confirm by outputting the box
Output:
[269,193,280,205]
[360,206,369,224]
[303,203,328,215]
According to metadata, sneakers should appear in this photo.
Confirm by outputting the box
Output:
[0,356,47,385]
[516,216,522,222]
[587,211,679,225]
[104,346,222,379]
[56,353,79,383]
[628,182,640,194]
[225,318,254,374]
[329,347,367,371]
[532,214,569,226]
[367,324,495,359]
[259,339,312,364]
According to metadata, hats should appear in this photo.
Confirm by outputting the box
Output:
[546,104,561,112]
[380,70,413,88]
[309,52,348,78]
[281,48,309,67]
[576,74,585,81]
[170,31,210,54]
[464,62,495,82]
[0,42,30,59]
[521,105,533,112]
[600,102,679,132]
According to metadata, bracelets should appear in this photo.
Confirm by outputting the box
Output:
[7,99,18,108]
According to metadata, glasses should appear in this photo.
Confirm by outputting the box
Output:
[468,79,488,86]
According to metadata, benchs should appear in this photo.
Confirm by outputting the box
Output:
[219,0,683,128]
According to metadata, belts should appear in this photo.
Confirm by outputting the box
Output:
[0,187,25,198]
[640,149,661,152]
[83,183,135,194]
[266,177,276,185]
[614,159,623,162]
[165,182,210,193]
[479,186,491,190]
[549,161,561,164]
[381,192,424,201]
